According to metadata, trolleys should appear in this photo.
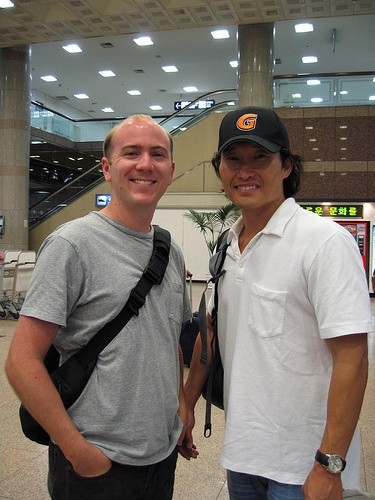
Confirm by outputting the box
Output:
[0,249,37,319]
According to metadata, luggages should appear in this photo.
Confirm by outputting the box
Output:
[179,273,200,368]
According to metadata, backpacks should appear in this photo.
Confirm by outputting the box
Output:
[198,228,229,408]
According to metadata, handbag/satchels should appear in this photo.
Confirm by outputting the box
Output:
[18,223,172,445]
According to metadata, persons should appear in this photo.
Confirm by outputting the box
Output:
[6,114,183,500]
[177,106,371,500]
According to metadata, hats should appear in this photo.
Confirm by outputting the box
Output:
[217,106,289,156]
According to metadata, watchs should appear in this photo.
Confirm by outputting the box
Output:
[314,449,346,475]
